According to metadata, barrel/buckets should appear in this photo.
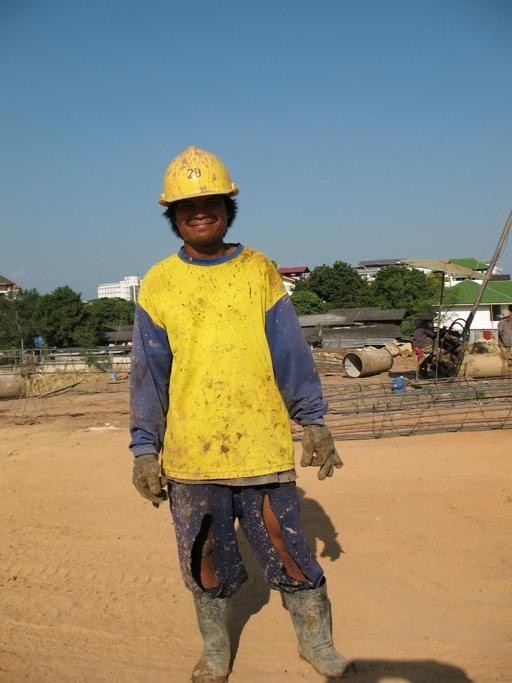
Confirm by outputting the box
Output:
[393,374,403,392]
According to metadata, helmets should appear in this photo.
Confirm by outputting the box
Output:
[158,147,240,205]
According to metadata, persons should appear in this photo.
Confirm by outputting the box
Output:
[414,312,436,348]
[128,146,356,683]
[497,309,512,348]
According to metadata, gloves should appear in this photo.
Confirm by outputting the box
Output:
[132,453,170,507]
[300,424,343,482]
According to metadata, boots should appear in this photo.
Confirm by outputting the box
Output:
[191,595,233,682]
[282,575,354,679]
